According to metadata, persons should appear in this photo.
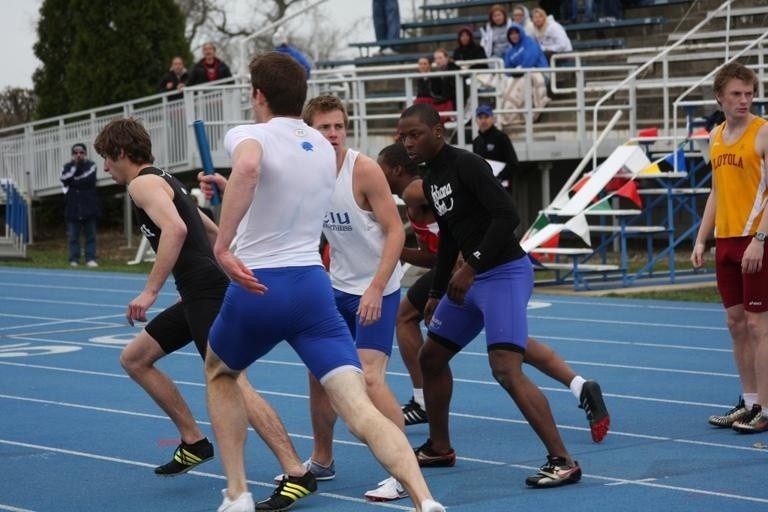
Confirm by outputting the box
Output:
[512,5,537,41]
[532,7,573,66]
[504,24,551,87]
[480,4,512,59]
[160,56,188,91]
[188,43,233,84]
[59,143,98,268]
[197,51,444,512]
[378,144,610,445]
[94,117,318,510]
[432,48,470,109]
[416,55,433,98]
[690,62,768,433]
[472,104,518,195]
[452,27,488,70]
[273,96,412,501]
[398,102,582,488]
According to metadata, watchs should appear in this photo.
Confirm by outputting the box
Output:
[752,231,767,242]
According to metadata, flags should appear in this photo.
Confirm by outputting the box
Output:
[525,128,712,264]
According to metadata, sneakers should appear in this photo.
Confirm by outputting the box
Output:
[707,395,748,426]
[255,470,318,512]
[364,475,409,501]
[154,435,215,476]
[420,499,447,512]
[86,259,99,268]
[216,488,255,512]
[731,403,768,435]
[413,438,456,468]
[401,395,429,426]
[273,456,336,484]
[577,380,611,443]
[524,454,583,488]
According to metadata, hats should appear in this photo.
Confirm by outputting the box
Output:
[475,104,493,116]
